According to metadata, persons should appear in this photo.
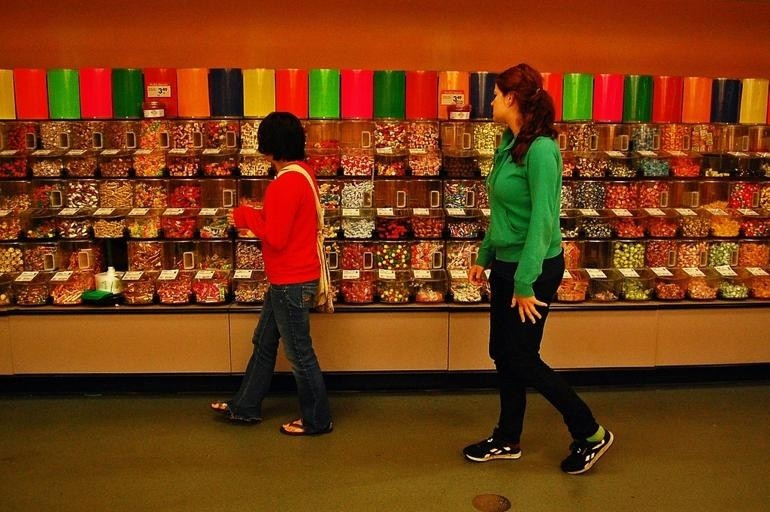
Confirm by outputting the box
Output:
[460,60,616,478]
[208,110,335,438]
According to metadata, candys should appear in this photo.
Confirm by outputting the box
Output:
[552,125,770,308]
[0,118,235,304]
[234,120,509,308]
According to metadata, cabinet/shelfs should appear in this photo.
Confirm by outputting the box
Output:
[0,118,770,400]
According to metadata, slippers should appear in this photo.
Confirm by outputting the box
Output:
[209,401,259,425]
[280,419,333,436]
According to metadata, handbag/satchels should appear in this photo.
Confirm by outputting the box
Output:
[310,235,334,313]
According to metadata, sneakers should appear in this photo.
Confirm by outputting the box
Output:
[462,436,522,463]
[560,426,615,475]
